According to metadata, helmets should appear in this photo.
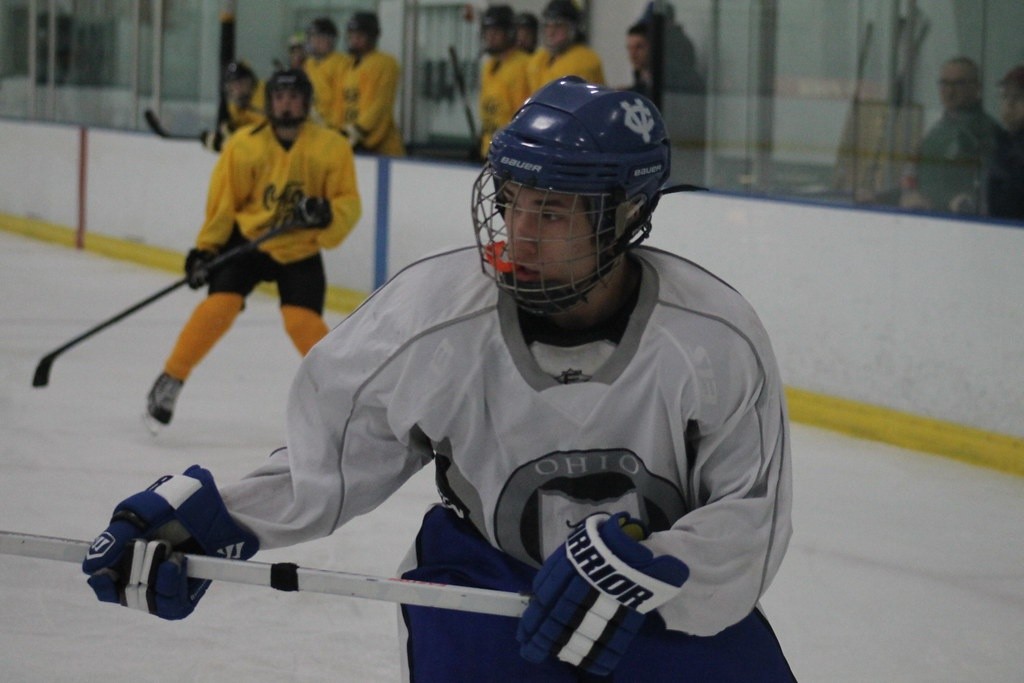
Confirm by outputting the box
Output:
[514,12,537,53]
[479,6,517,54]
[344,11,380,55]
[223,61,258,108]
[307,18,338,60]
[541,0,579,54]
[485,76,672,318]
[264,69,315,123]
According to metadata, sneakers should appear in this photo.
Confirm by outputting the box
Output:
[142,371,184,436]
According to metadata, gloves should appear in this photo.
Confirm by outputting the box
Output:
[513,510,691,676]
[296,193,334,230]
[184,247,216,289]
[81,464,260,620]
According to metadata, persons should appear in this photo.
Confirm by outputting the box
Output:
[83,68,800,683]
[472,0,707,173]
[196,9,407,161]
[898,57,1024,217]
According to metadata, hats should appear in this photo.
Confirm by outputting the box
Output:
[994,65,1024,87]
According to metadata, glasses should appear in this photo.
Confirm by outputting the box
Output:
[938,78,974,85]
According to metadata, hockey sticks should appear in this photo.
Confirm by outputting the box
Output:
[447,44,484,162]
[0,531,530,618]
[33,226,286,391]
[144,107,204,141]
[837,0,931,205]
